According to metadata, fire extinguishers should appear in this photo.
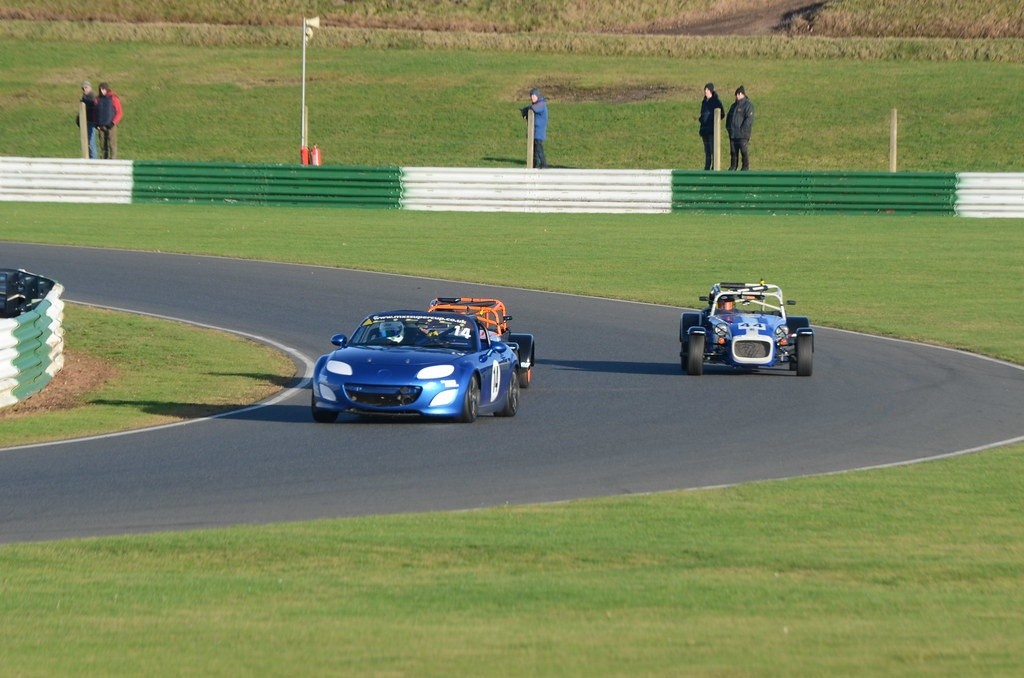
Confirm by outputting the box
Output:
[300,146,312,165]
[310,144,321,166]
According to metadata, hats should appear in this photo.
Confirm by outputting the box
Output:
[529,88,540,98]
[735,85,745,96]
[99,83,108,91]
[81,80,92,89]
[705,83,714,94]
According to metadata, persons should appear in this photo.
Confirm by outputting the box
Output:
[726,84,754,170]
[92,82,122,159]
[714,295,746,314]
[522,86,548,168]
[368,321,432,345]
[76,81,98,159]
[699,83,725,170]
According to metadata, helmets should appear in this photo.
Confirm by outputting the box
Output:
[718,295,735,312]
[379,321,405,343]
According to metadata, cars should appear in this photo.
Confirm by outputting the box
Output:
[312,310,520,423]
[679,277,815,375]
[412,297,535,388]
